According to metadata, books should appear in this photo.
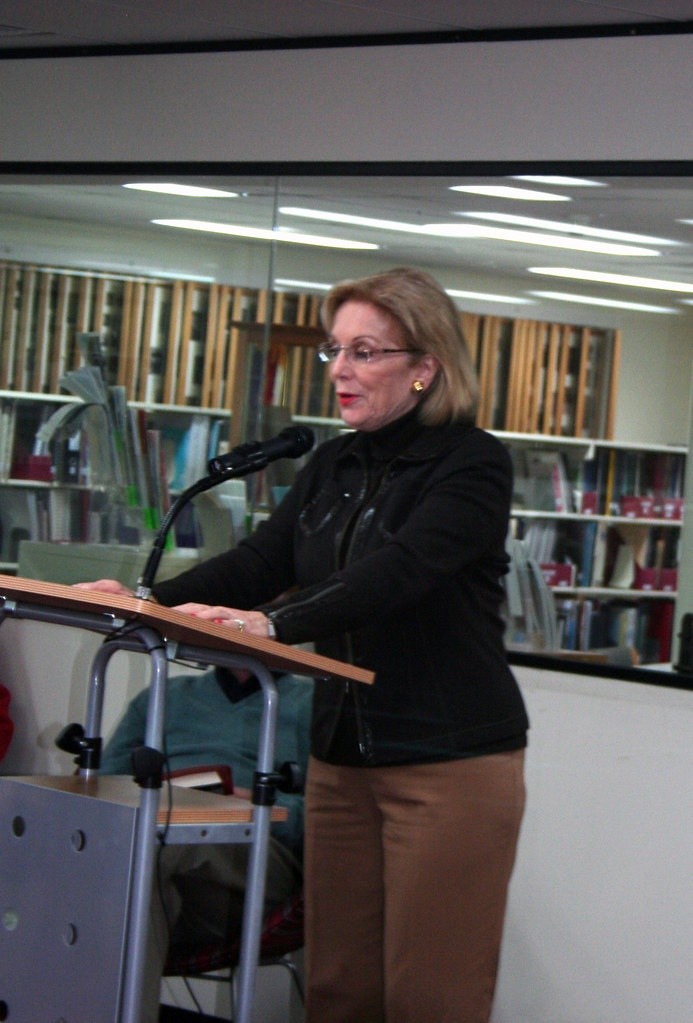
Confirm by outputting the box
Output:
[0,334,327,546]
[501,448,686,663]
[164,771,226,796]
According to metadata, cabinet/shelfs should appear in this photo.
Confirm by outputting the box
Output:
[1,390,688,676]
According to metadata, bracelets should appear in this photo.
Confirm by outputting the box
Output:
[269,619,276,638]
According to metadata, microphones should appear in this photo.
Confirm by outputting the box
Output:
[207,425,315,478]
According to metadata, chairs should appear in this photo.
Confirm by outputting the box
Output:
[158,950,303,1023]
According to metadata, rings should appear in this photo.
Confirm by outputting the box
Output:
[232,619,245,632]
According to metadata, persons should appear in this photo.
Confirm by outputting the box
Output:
[98,588,313,976]
[73,265,531,1023]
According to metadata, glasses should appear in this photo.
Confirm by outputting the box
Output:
[319,340,424,364]
